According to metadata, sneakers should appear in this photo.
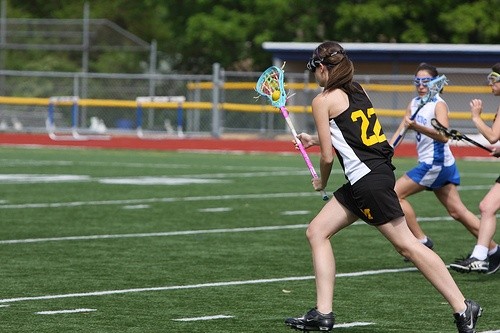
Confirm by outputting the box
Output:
[488,247,500,275]
[449,256,489,273]
[403,237,432,262]
[284,309,334,332]
[453,299,482,333]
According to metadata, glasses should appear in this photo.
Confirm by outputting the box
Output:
[487,72,500,83]
[306,58,319,73]
[413,78,437,86]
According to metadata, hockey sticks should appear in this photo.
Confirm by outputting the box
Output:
[430,117,499,157]
[255,67,330,202]
[392,74,447,149]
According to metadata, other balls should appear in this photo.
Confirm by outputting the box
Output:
[272,91,280,100]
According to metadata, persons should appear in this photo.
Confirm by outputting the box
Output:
[450,64,500,274]
[285,41,483,333]
[389,63,500,275]
